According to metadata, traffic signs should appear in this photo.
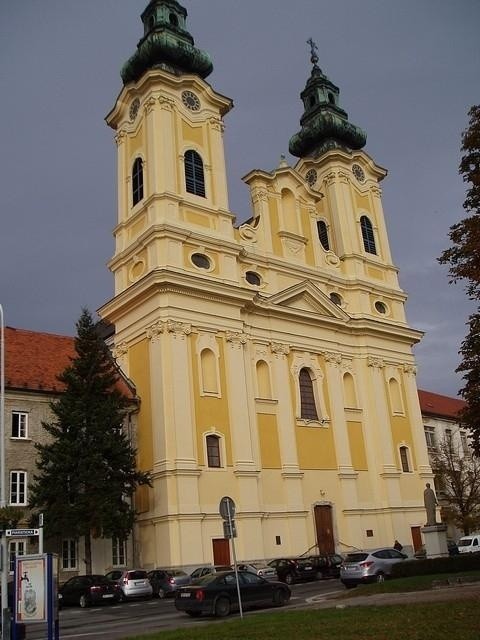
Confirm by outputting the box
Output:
[12,529,34,535]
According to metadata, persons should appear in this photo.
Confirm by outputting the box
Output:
[424,483,439,524]
